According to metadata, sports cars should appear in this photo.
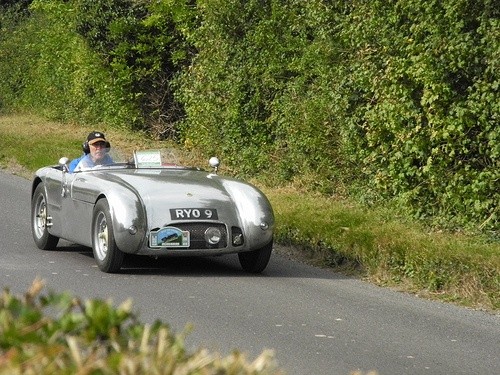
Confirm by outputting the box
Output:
[29,148,275,274]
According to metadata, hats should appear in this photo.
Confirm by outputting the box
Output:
[87,131,106,144]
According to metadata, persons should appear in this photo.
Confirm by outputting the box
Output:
[70,131,113,171]
